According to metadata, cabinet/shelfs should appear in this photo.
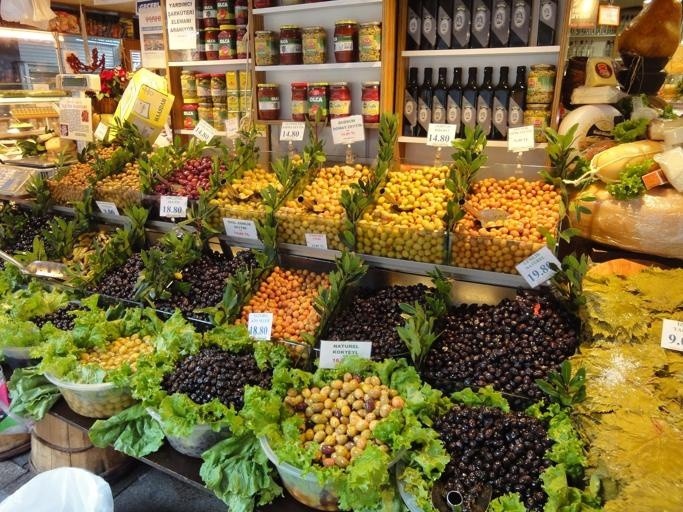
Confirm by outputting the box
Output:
[0,20,133,107]
[158,0,256,159]
[245,0,396,166]
[387,0,571,171]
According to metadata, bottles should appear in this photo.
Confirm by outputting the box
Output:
[405,69,418,138]
[180,71,195,98]
[258,83,278,119]
[528,63,551,103]
[198,101,213,127]
[303,28,324,64]
[203,29,215,61]
[308,83,326,122]
[215,2,229,24]
[236,26,247,57]
[478,66,492,138]
[526,106,546,142]
[256,30,274,65]
[182,101,195,131]
[508,64,525,131]
[419,65,430,138]
[448,66,461,138]
[464,66,480,138]
[358,22,380,63]
[361,82,379,123]
[202,5,211,24]
[291,82,307,122]
[333,20,357,63]
[329,82,350,120]
[219,27,232,60]
[280,26,298,64]
[211,74,226,102]
[493,66,511,141]
[234,3,244,23]
[433,68,448,125]
[195,75,211,102]
[213,102,226,131]
